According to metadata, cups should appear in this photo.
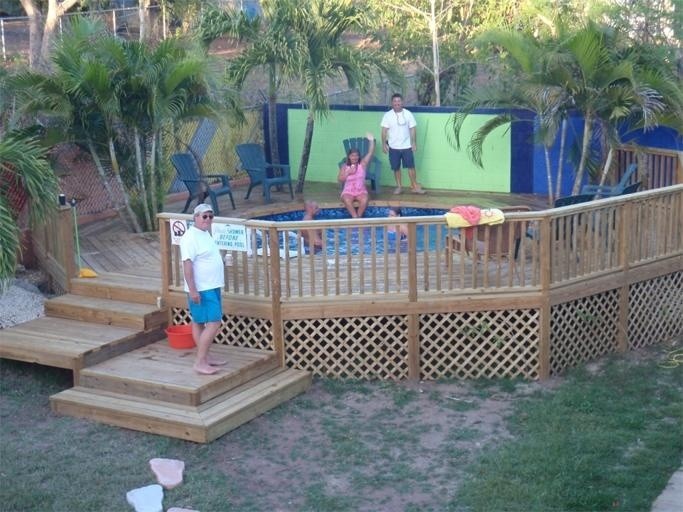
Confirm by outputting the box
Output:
[59,193,66,204]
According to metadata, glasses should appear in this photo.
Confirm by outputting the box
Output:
[201,215,213,219]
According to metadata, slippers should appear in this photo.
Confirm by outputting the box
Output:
[411,188,426,193]
[394,187,402,194]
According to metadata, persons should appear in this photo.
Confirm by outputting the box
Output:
[180,202,228,376]
[380,93,426,196]
[381,208,408,245]
[336,132,376,219]
[299,197,323,254]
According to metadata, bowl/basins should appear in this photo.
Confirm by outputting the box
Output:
[164,325,198,349]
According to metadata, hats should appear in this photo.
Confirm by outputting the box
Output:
[193,203,213,214]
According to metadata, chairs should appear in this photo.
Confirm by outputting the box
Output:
[170,141,295,215]
[445,163,644,270]
[338,138,380,195]
